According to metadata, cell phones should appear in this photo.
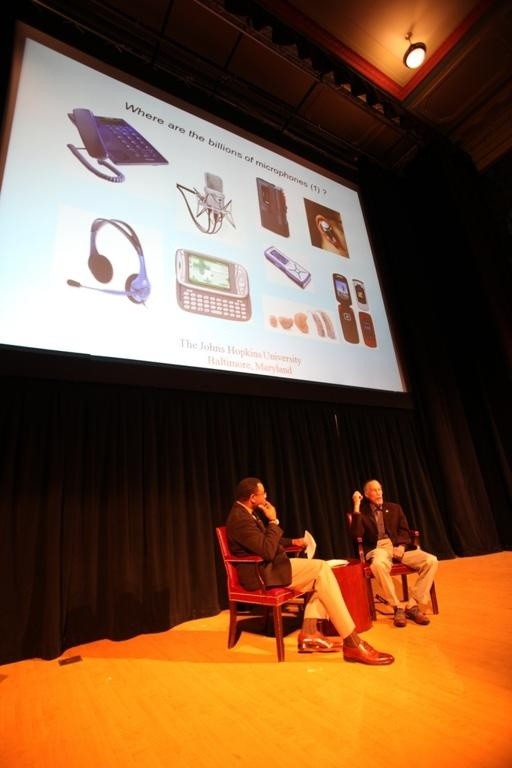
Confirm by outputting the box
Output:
[332,273,360,344]
[352,278,378,349]
[175,249,252,321]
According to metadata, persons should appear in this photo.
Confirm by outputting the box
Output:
[350,480,438,626]
[226,477,394,665]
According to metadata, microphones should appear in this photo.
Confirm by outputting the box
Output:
[204,172,225,222]
[67,279,130,297]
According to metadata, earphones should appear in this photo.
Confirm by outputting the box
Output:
[320,220,333,235]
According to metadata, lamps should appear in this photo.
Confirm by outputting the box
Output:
[402,29,427,70]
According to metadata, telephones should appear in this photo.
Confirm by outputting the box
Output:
[68,108,170,166]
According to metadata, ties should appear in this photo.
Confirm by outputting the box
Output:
[252,511,266,534]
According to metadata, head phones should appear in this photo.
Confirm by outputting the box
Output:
[88,217,151,304]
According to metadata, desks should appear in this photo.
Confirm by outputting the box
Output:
[316,559,373,637]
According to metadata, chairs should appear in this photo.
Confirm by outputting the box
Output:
[346,511,439,621]
[215,525,323,662]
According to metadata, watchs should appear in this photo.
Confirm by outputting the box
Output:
[268,519,280,525]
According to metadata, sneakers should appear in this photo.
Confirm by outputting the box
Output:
[394,607,408,627]
[405,604,430,625]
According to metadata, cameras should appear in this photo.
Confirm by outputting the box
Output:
[256,177,290,237]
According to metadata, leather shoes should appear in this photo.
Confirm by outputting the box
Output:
[298,632,344,652]
[343,639,395,666]
